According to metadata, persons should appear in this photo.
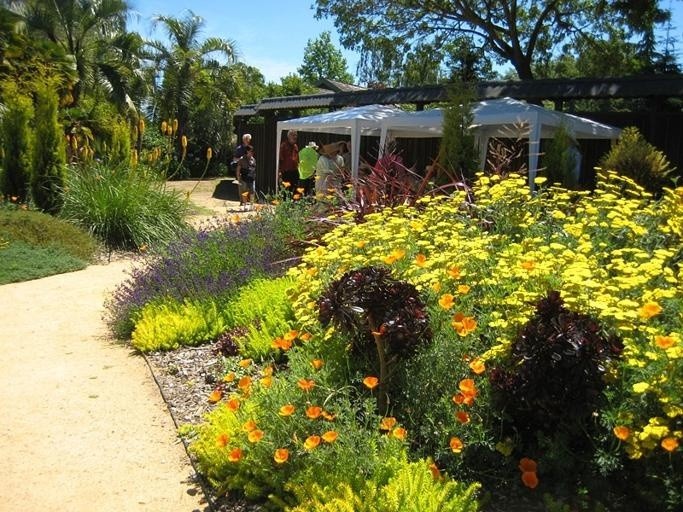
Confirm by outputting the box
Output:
[297,142,319,183]
[278,130,298,189]
[336,141,349,165]
[236,146,256,206]
[233,133,253,163]
[315,144,345,194]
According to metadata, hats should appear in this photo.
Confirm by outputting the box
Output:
[306,142,319,150]
[319,144,339,155]
